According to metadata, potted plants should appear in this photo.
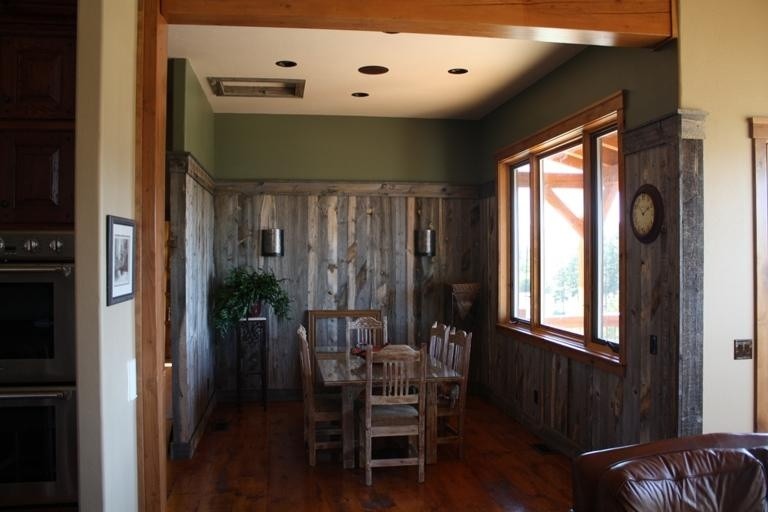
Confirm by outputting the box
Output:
[209,263,298,344]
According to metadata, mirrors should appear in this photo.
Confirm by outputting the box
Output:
[308,310,384,351]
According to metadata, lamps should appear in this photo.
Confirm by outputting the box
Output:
[414,227,437,255]
[260,227,285,257]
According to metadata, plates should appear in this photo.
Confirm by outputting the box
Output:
[351,343,389,360]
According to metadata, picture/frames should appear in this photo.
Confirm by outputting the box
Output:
[105,212,137,308]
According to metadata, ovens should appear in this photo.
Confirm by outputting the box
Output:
[1,230,77,511]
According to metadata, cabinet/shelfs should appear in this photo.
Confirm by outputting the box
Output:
[0,0,76,229]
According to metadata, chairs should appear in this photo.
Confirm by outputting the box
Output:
[570,432,768,510]
[295,316,474,488]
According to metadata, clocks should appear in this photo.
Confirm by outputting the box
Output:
[630,182,663,244]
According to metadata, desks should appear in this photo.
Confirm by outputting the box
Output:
[236,316,270,417]
